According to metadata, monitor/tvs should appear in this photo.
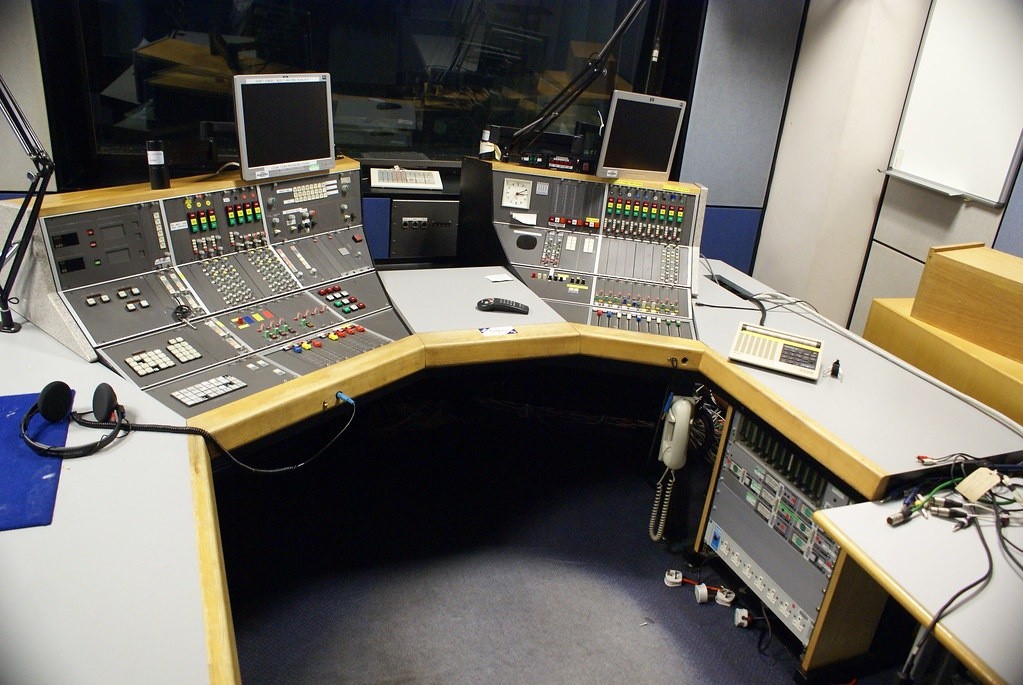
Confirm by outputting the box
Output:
[477,22,549,89]
[232,73,335,182]
[595,90,687,181]
[252,0,316,72]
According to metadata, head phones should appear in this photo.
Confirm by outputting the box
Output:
[19,381,125,459]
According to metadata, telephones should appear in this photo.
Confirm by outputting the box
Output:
[657,395,695,471]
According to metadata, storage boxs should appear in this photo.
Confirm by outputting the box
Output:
[911,241,1023,363]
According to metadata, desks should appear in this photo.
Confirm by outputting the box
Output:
[131,35,633,138]
[0,150,1023,685]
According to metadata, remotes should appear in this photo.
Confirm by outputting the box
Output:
[477,297,529,314]
[376,102,402,110]
